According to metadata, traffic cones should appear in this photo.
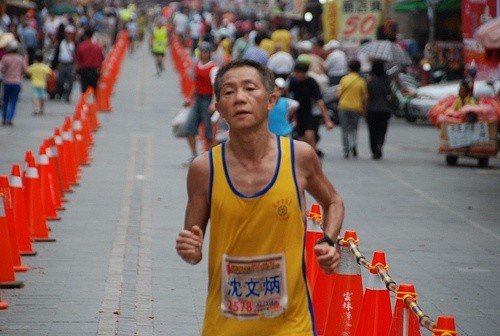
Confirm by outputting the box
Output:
[49,27,129,204]
[8,162,28,272]
[303,203,325,293]
[43,136,66,211]
[389,283,422,336]
[36,144,61,220]
[433,316,456,336]
[326,230,363,336]
[311,256,332,336]
[354,250,393,336]
[0,174,24,288]
[164,26,220,156]
[21,149,57,242]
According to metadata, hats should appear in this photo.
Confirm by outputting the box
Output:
[199,41,212,50]
[322,40,339,50]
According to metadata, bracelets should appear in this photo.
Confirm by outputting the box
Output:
[317,238,342,254]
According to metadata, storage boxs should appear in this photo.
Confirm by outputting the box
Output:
[441,120,497,150]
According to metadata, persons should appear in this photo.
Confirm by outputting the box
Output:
[0,0,458,160]
[176,59,344,336]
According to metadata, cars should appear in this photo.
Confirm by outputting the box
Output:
[405,72,499,125]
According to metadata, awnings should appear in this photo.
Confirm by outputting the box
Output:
[393,0,462,11]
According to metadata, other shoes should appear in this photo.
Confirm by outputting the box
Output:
[345,155,349,158]
[32,110,42,115]
[352,146,357,156]
[2,120,10,125]
[183,152,198,166]
[373,145,382,159]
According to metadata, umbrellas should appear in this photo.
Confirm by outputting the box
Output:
[359,40,412,65]
[48,4,77,14]
